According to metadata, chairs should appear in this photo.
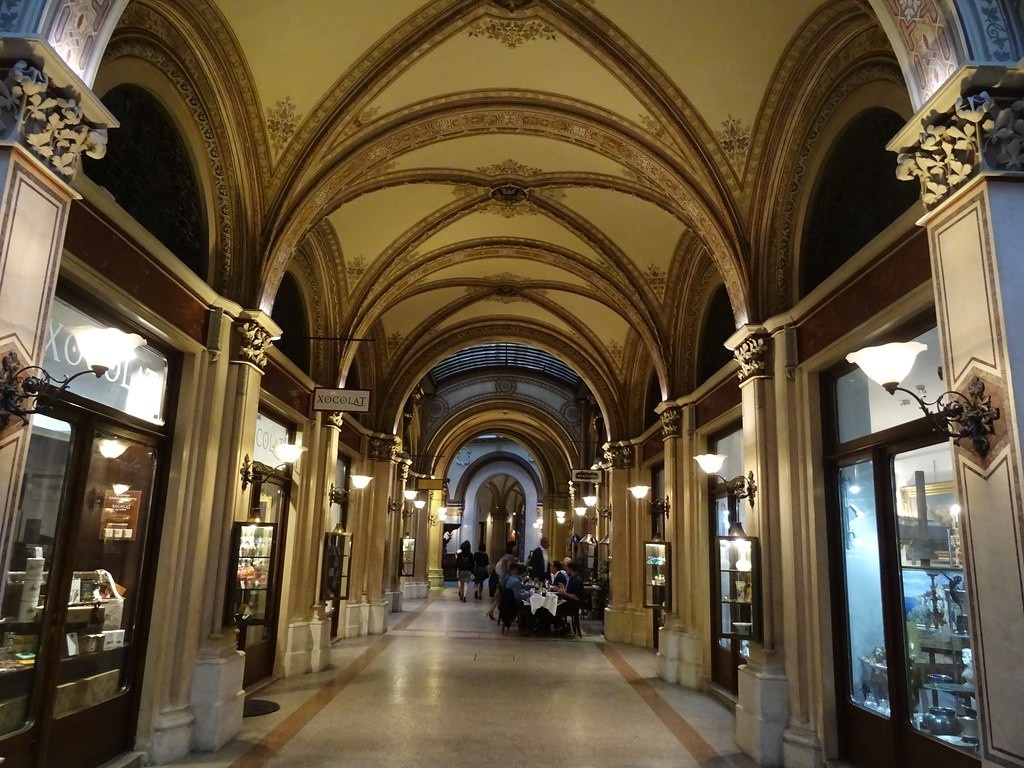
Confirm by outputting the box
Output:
[497,582,583,639]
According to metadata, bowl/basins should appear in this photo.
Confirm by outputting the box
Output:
[732,622,752,635]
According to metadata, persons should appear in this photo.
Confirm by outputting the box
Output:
[939,570,964,615]
[961,648,973,686]
[869,646,888,708]
[909,656,924,721]
[455,536,584,638]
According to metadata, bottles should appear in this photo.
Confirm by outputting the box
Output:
[542,582,546,597]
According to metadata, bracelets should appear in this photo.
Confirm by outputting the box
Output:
[566,593,568,596]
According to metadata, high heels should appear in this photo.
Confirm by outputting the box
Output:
[486,613,496,620]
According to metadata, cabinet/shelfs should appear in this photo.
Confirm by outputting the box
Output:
[0,568,131,740]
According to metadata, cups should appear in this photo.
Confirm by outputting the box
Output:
[18,558,45,623]
[736,581,745,603]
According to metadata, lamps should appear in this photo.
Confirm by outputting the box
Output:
[692,454,756,510]
[626,485,671,520]
[388,491,417,516]
[402,500,427,522]
[555,511,574,533]
[428,507,448,527]
[329,473,373,508]
[239,443,308,492]
[583,496,612,522]
[845,337,1000,461]
[572,507,598,526]
[0,324,148,437]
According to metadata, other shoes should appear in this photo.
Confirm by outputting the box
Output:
[460,595,462,601]
[463,597,466,602]
[475,591,479,599]
[480,596,482,600]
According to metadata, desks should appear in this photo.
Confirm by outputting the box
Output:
[523,580,566,638]
